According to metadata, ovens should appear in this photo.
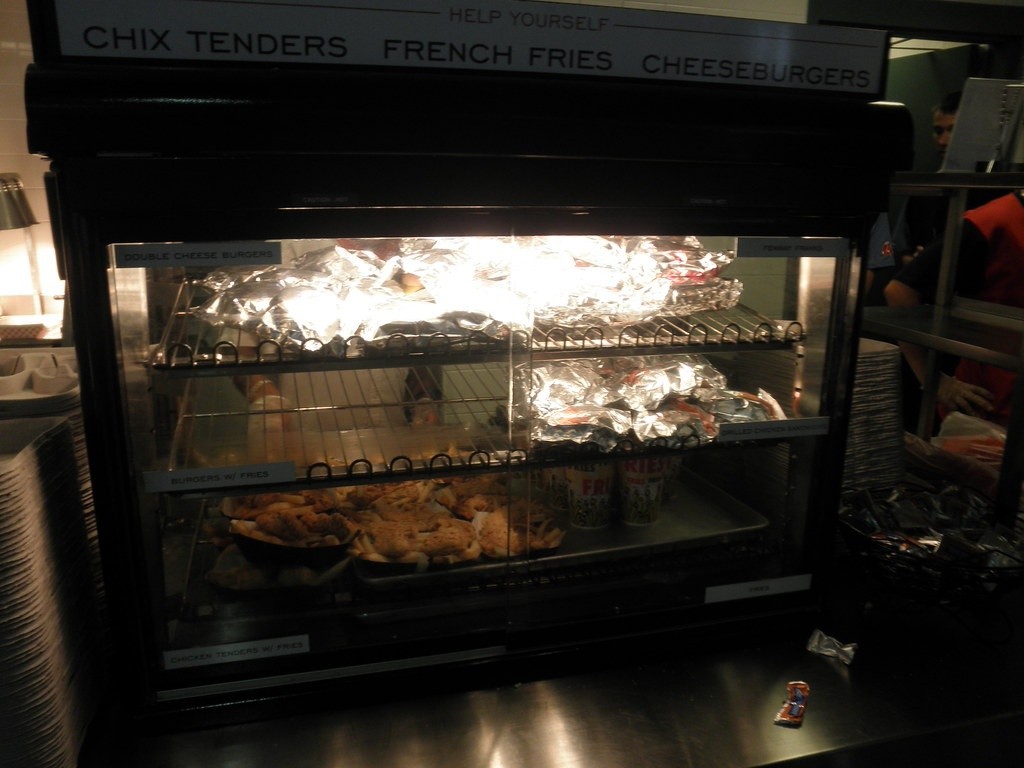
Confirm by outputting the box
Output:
[20,1,910,741]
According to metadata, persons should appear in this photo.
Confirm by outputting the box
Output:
[881,88,987,442]
[886,184,1022,453]
[860,195,901,357]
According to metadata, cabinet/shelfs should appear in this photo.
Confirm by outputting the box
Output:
[0,146,1024,743]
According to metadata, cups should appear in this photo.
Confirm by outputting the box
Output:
[566,462,613,529]
[541,466,569,511]
[661,456,680,497]
[615,459,663,527]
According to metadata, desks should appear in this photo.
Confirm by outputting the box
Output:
[76,598,1024,768]
